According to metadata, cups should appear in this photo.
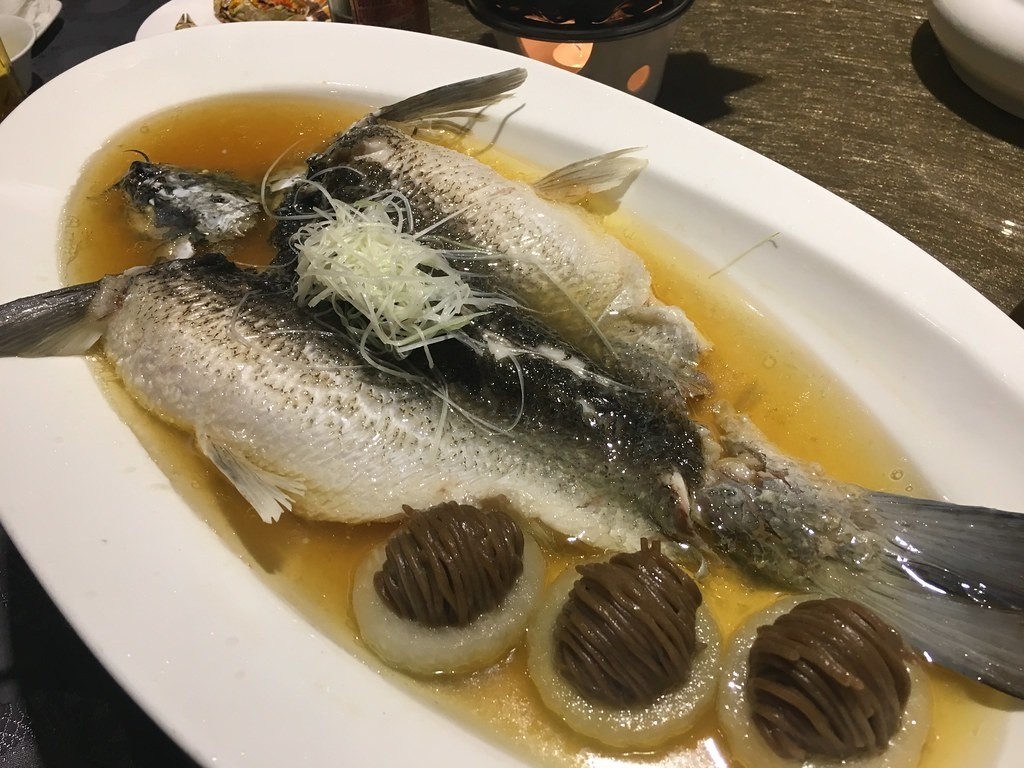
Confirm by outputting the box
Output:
[0,14,36,91]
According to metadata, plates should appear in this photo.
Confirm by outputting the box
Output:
[0,22,1024,768]
[134,0,225,39]
[1,0,64,41]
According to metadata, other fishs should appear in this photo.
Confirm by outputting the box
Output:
[0,66,1024,703]
